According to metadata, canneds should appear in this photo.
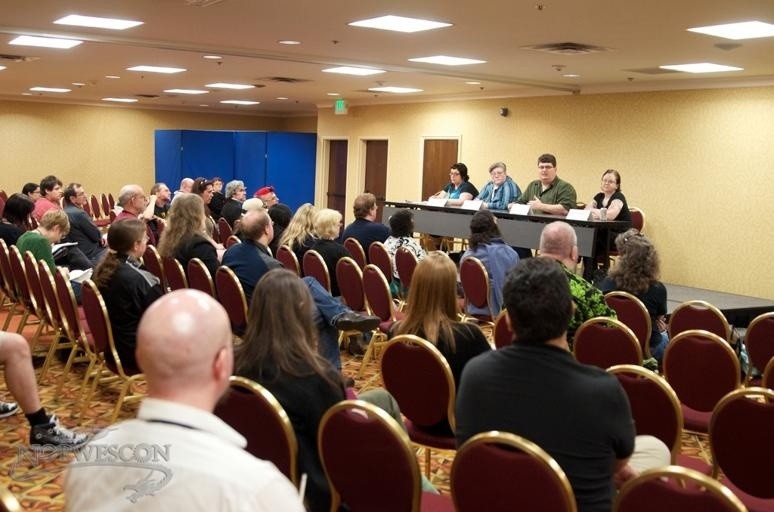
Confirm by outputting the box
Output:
[600,208,607,221]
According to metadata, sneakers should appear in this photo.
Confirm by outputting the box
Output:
[29,414,89,452]
[1,400,18,419]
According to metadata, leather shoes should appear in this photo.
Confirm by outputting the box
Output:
[335,312,381,331]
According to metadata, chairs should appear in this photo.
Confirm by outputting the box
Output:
[0,189,774,512]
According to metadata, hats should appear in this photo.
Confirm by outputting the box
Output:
[254,185,274,197]
